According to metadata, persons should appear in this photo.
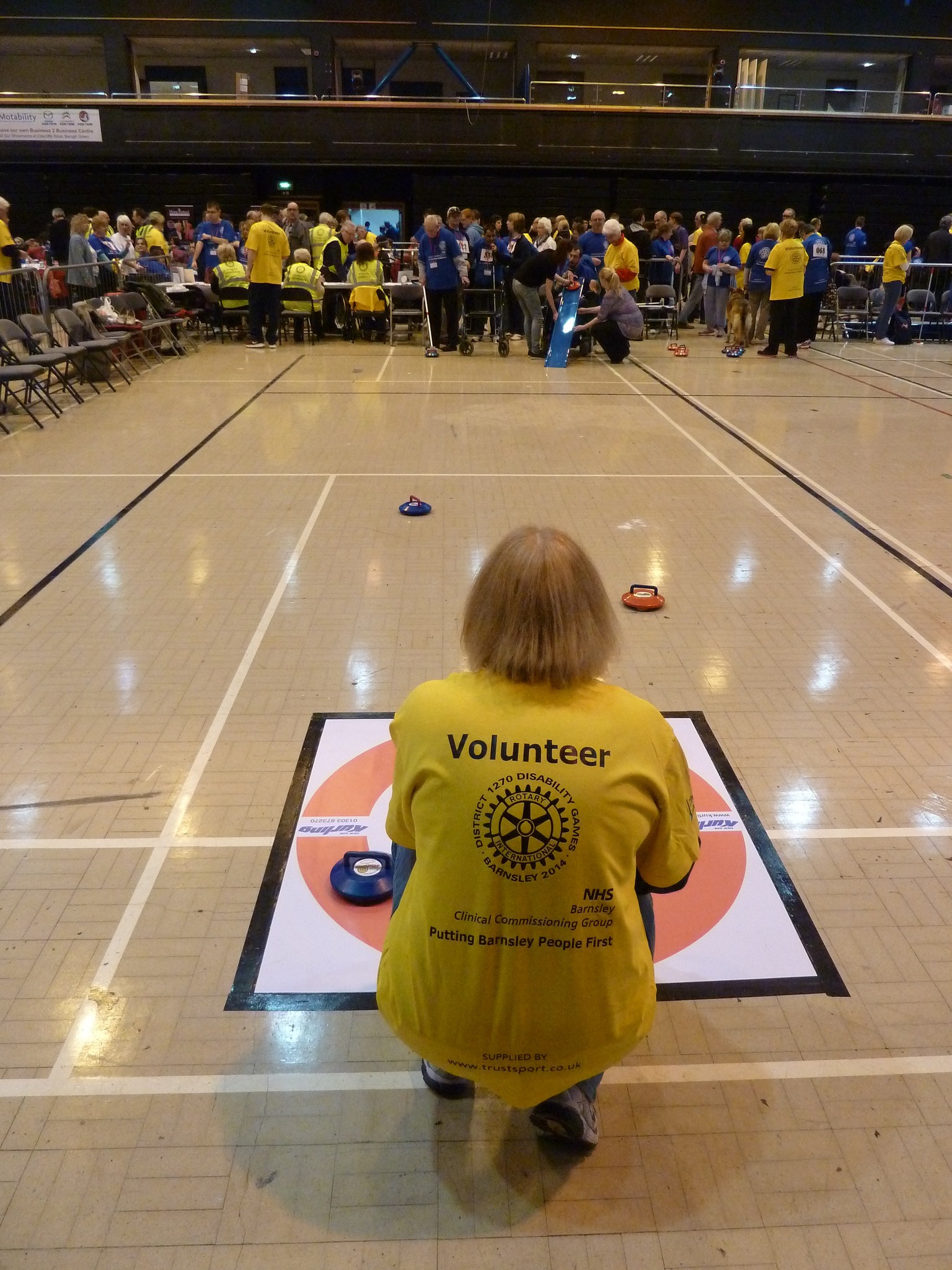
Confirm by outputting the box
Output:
[0,197,951,356]
[383,529,704,1142]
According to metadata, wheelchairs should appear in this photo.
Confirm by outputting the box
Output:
[540,282,597,355]
[459,280,511,357]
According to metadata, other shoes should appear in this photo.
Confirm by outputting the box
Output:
[594,346,609,354]
[161,345,187,356]
[293,333,325,343]
[426,343,457,352]
[677,324,695,330]
[668,327,678,333]
[504,333,523,341]
[758,348,779,358]
[470,335,483,342]
[796,339,812,350]
[528,349,547,358]
[326,326,342,334]
[583,350,595,357]
[699,329,715,336]
[873,336,895,347]
[117,307,136,325]
[717,330,726,338]
[649,328,656,334]
[214,327,223,334]
[268,342,277,349]
[753,336,770,344]
[786,351,798,358]
[245,339,265,349]
[612,342,630,364]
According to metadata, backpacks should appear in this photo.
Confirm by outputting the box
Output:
[889,306,913,345]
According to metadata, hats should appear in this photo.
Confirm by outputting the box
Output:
[447,207,461,216]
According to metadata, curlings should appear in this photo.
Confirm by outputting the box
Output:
[330,850,393,904]
[722,346,746,358]
[566,281,580,291]
[621,584,665,612]
[667,343,689,357]
[399,495,431,516]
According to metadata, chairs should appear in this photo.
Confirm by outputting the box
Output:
[641,285,678,340]
[813,286,952,345]
[0,285,424,435]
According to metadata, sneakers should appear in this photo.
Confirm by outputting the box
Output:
[420,1056,468,1095]
[526,1084,599,1147]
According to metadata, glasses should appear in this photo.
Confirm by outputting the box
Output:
[208,211,218,214]
[782,215,793,218]
[345,229,356,236]
[532,222,538,224]
[286,208,299,211]
[537,227,544,229]
[818,224,822,226]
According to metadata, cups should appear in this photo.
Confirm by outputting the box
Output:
[39,261,44,268]
[34,260,39,263]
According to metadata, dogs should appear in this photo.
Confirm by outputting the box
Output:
[724,286,752,347]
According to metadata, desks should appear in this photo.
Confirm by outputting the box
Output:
[154,280,425,334]
